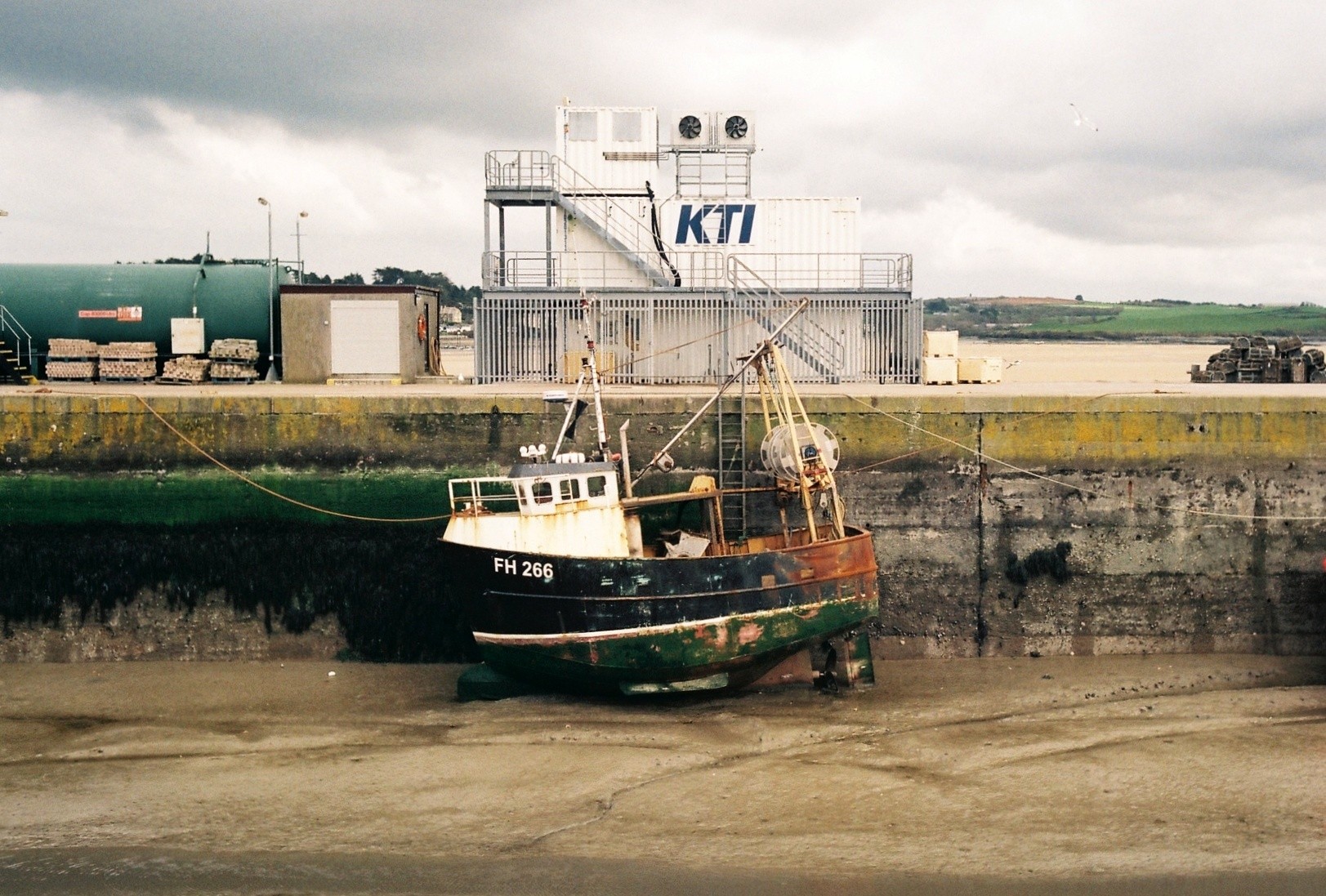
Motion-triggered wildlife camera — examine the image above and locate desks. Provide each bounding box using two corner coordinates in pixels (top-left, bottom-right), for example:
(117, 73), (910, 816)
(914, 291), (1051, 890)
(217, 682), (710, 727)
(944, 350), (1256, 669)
(563, 352), (614, 384)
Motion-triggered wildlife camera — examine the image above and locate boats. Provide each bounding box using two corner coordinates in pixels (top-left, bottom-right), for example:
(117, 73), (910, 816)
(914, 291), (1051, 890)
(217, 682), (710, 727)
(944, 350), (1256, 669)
(432, 283), (881, 704)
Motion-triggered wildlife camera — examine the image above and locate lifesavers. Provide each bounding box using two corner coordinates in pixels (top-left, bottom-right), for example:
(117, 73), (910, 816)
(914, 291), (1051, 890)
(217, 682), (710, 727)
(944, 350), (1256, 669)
(417, 313), (426, 342)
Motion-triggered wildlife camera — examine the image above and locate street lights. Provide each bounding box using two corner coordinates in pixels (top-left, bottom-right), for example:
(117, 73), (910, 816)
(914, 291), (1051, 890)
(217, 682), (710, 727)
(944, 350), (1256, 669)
(296, 211), (308, 286)
(257, 195), (281, 383)
